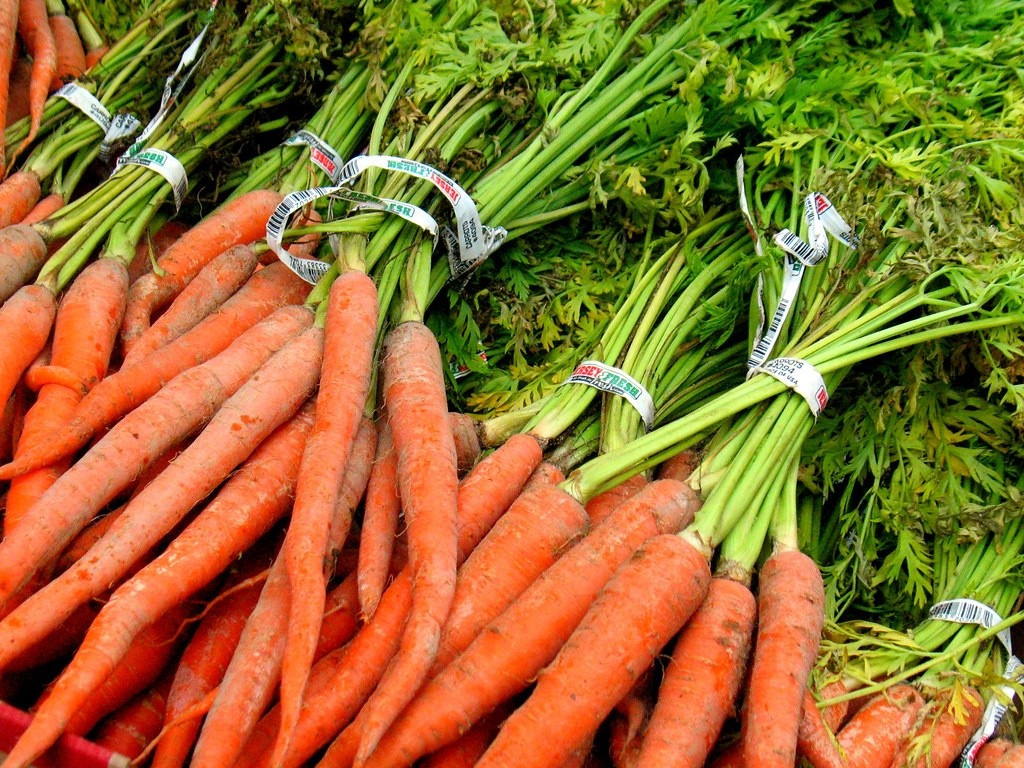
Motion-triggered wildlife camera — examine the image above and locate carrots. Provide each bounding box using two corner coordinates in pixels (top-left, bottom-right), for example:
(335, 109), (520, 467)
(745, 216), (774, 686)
(0, 0), (1024, 768)
